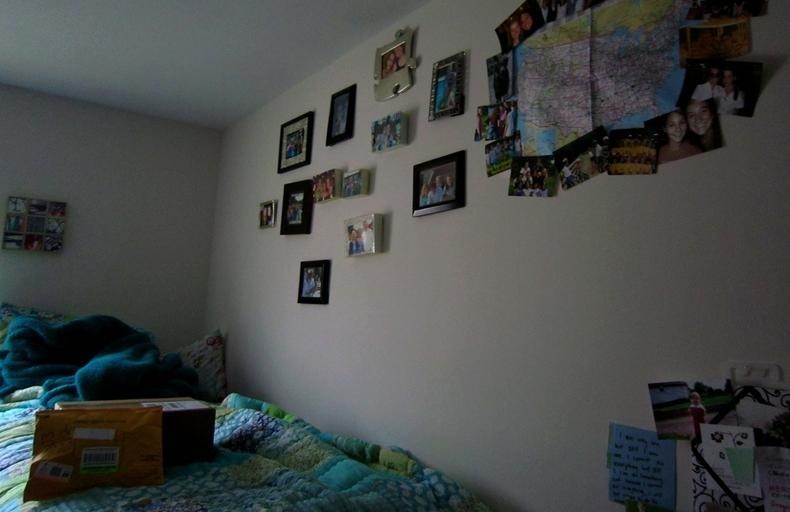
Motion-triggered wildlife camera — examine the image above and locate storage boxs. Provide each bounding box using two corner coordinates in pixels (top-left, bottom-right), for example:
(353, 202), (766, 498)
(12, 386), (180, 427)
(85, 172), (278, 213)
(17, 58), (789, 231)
(54, 397), (215, 464)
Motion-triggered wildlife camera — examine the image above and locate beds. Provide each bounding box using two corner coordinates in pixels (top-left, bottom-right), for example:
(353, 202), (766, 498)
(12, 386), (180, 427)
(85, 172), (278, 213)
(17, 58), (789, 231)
(0, 303), (514, 510)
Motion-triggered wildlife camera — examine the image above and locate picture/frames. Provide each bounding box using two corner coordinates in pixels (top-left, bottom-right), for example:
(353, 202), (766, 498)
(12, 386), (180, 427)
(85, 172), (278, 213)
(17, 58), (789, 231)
(428, 45), (470, 123)
(372, 27), (417, 102)
(297, 261), (331, 305)
(256, 201), (276, 228)
(324, 84), (358, 145)
(413, 150), (467, 218)
(272, 112), (314, 176)
(281, 179), (315, 236)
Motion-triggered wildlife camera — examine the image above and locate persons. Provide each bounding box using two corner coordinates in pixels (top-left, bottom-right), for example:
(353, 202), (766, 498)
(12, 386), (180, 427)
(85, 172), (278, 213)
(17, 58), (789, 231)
(561, 101), (714, 185)
(287, 205), (302, 221)
(474, 58), (520, 164)
(349, 220), (373, 255)
(382, 46), (405, 77)
(689, 1), (749, 114)
(514, 159), (548, 197)
(303, 269), (321, 298)
(444, 65), (456, 108)
(420, 176), (454, 206)
(508, 1), (580, 51)
(688, 391), (705, 435)
(262, 207), (272, 225)
